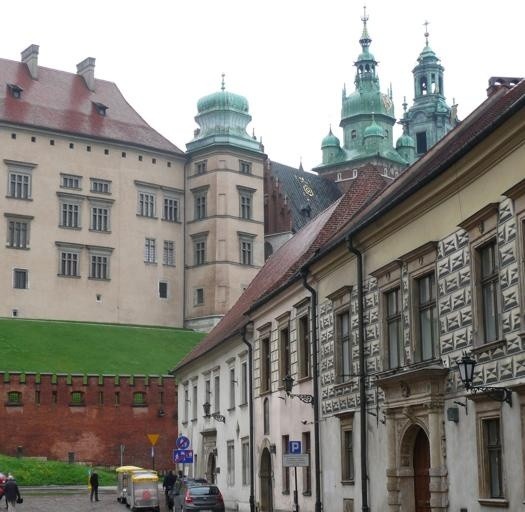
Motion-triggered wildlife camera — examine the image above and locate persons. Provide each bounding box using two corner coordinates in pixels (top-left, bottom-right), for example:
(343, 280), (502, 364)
(0, 472), (20, 512)
(90, 469), (100, 502)
(163, 470), (177, 505)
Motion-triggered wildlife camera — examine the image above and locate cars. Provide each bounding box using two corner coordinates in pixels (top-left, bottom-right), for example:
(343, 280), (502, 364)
(168, 477), (225, 512)
(0, 473), (8, 499)
